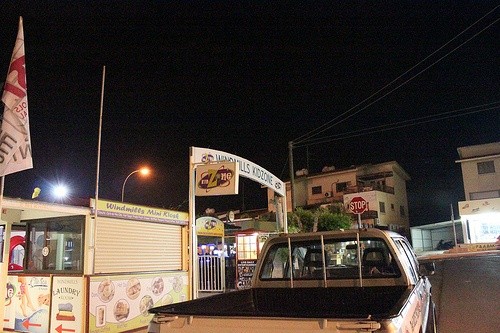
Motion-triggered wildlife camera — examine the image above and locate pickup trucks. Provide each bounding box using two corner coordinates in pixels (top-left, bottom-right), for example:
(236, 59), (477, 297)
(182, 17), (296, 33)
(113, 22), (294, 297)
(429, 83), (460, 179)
(147, 228), (438, 333)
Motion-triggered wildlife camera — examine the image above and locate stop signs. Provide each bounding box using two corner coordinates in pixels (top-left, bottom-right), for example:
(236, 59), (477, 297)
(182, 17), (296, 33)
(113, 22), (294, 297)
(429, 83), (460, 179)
(350, 198), (367, 215)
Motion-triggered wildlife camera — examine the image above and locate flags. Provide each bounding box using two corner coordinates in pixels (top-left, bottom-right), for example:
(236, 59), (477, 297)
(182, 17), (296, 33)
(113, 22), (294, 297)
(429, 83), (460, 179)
(0, 15), (34, 177)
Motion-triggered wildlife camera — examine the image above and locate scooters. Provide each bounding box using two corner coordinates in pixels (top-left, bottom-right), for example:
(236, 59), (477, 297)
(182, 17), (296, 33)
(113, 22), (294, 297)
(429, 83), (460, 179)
(436, 240), (452, 250)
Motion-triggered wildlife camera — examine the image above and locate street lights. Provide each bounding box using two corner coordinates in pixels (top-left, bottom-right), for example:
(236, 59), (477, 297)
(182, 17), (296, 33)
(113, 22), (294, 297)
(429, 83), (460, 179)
(121, 167), (149, 202)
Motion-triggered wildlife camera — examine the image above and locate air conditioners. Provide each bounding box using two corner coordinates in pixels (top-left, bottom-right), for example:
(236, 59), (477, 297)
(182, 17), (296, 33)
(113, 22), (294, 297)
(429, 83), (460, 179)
(388, 223), (400, 232)
(324, 190), (333, 197)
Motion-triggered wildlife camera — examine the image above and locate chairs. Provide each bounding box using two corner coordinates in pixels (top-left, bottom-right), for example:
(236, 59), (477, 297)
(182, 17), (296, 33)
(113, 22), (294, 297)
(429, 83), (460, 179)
(302, 248), (329, 279)
(360, 247), (391, 278)
